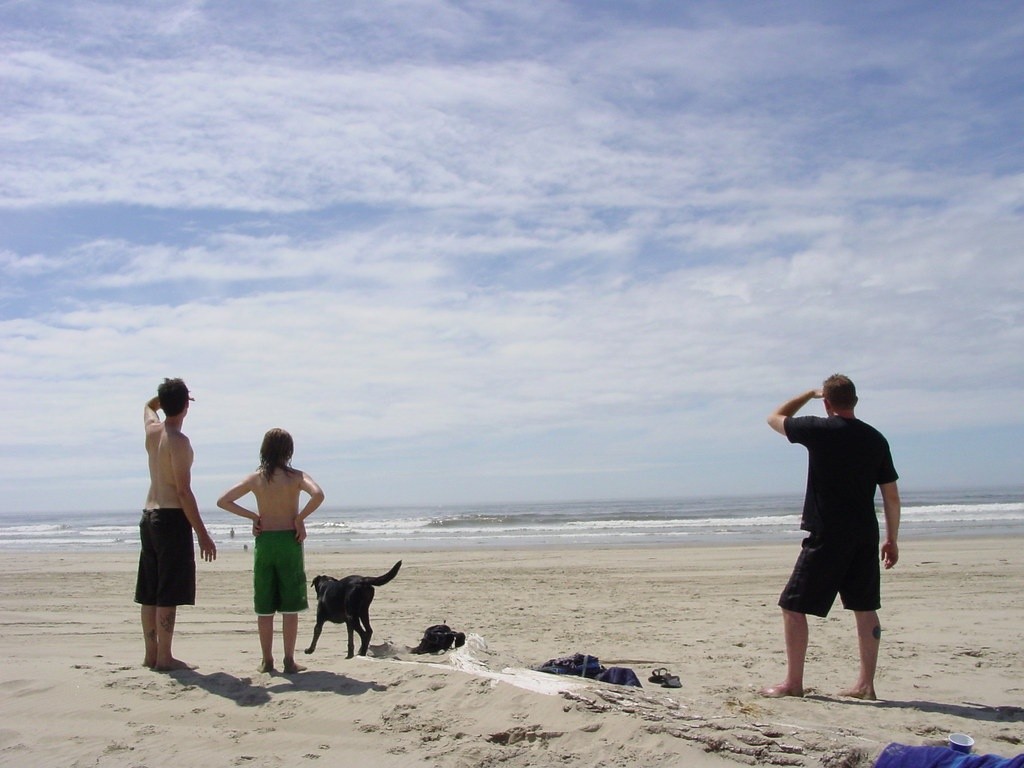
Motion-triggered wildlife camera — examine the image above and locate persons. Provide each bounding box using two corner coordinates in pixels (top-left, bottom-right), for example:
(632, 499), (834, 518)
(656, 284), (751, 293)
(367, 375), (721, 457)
(230, 527), (234, 537)
(134, 377), (216, 670)
(759, 373), (900, 703)
(217, 428), (325, 675)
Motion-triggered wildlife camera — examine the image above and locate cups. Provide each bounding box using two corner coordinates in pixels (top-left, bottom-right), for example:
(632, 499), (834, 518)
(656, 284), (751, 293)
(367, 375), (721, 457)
(948, 733), (975, 755)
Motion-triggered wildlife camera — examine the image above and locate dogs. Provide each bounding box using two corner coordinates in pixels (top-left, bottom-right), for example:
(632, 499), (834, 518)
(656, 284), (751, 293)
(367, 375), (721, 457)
(304, 558), (402, 660)
